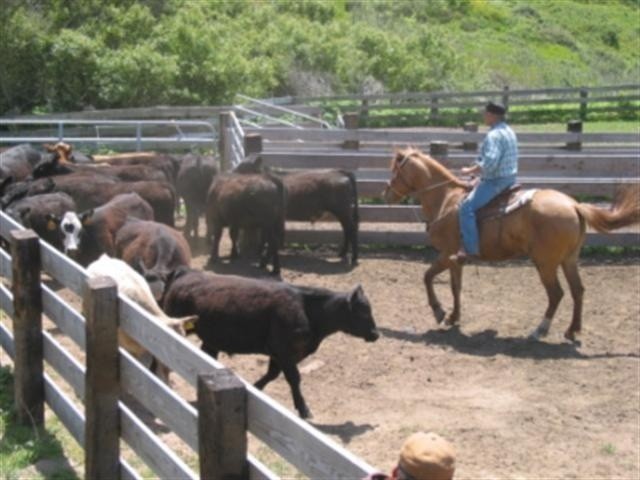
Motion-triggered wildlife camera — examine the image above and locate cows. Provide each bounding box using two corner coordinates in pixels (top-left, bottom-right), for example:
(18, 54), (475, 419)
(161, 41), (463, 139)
(82, 252), (199, 386)
(150, 262), (379, 421)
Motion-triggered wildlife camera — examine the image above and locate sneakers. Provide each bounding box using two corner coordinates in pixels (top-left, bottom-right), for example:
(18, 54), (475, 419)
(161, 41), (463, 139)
(448, 250), (483, 266)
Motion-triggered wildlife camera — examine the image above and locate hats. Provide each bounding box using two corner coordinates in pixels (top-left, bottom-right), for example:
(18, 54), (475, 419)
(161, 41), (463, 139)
(476, 98), (511, 121)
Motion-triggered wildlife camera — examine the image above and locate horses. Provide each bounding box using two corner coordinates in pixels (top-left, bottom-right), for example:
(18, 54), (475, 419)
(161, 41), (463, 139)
(204, 166), (280, 277)
(3, 143), (216, 300)
(262, 168), (359, 269)
(382, 142), (639, 344)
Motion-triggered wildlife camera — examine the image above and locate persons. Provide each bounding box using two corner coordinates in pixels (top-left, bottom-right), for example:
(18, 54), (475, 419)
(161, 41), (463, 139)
(447, 100), (521, 262)
